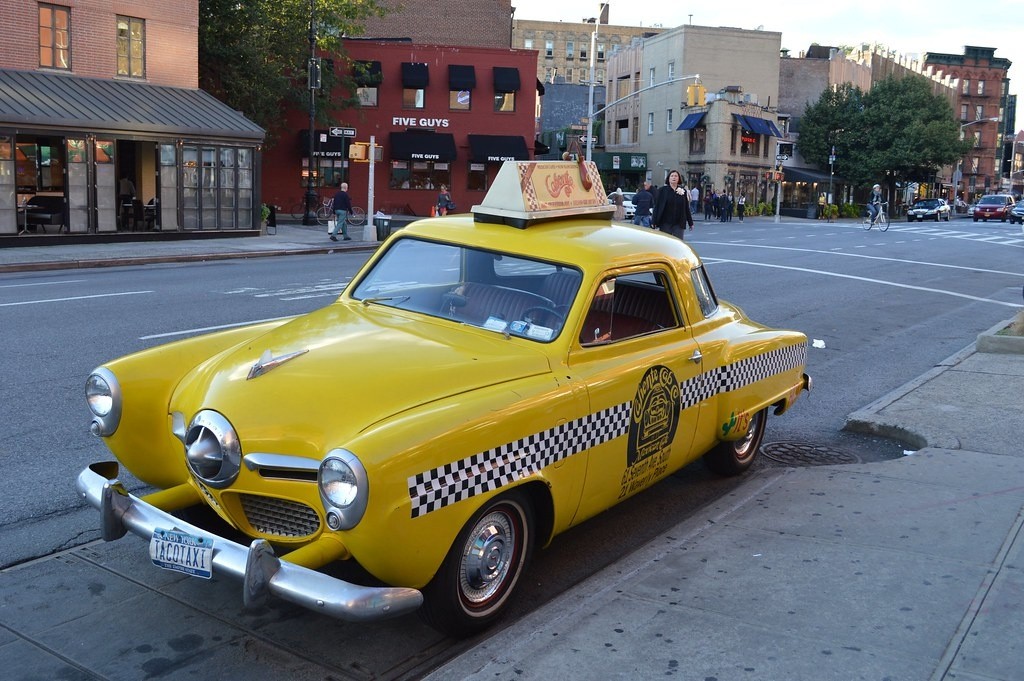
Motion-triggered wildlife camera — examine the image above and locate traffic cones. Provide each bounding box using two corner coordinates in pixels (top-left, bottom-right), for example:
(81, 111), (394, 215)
(430, 206), (435, 217)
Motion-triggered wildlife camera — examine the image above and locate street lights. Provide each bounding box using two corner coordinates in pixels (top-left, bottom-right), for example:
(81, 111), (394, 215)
(950, 117), (998, 215)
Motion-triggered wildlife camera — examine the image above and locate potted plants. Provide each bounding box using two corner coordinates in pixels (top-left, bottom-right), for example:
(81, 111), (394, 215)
(259, 206), (271, 235)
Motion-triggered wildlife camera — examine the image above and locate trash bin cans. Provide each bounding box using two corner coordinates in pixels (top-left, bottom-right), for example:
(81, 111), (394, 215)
(374, 216), (392, 240)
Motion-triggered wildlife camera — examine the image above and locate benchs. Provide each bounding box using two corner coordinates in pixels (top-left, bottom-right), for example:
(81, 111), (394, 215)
(541, 269), (674, 329)
(18, 196), (66, 234)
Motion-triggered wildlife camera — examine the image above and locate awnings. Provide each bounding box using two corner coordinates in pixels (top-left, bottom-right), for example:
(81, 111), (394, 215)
(537, 78), (545, 96)
(390, 132), (457, 161)
(352, 60), (382, 84)
(493, 67), (520, 91)
(449, 64), (476, 88)
(402, 62), (429, 87)
(468, 135), (530, 162)
(676, 112), (705, 131)
(735, 115), (782, 138)
(300, 129), (351, 158)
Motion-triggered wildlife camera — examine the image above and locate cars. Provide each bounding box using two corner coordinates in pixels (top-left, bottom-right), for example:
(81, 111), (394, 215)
(1010, 200), (1024, 224)
(950, 201), (969, 213)
(74, 158), (813, 641)
(967, 205), (975, 217)
(907, 197), (950, 222)
(607, 190), (652, 219)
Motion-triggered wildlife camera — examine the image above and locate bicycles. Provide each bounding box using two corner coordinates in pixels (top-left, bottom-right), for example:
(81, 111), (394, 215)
(861, 202), (890, 231)
(291, 194), (366, 226)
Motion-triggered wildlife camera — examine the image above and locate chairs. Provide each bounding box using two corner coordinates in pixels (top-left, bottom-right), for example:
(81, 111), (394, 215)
(443, 281), (555, 326)
(550, 305), (657, 344)
(117, 199), (156, 233)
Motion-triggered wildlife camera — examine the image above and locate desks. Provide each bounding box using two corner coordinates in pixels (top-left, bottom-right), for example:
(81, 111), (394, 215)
(122, 204), (156, 232)
(18, 204), (37, 235)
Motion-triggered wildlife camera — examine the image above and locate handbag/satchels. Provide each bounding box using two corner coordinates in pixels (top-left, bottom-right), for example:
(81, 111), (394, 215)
(327, 216), (343, 234)
(436, 210), (439, 217)
(447, 202), (456, 210)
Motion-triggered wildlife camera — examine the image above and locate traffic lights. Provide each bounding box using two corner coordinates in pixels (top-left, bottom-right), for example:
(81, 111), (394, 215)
(697, 86), (707, 106)
(765, 171), (774, 180)
(775, 172), (784, 182)
(368, 146), (383, 162)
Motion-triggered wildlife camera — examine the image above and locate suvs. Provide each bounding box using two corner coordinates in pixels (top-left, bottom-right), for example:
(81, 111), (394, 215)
(973, 194), (1016, 223)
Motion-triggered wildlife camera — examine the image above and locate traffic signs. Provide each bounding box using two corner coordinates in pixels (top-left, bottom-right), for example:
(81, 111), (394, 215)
(329, 127), (356, 138)
(776, 154), (789, 160)
(579, 136), (598, 144)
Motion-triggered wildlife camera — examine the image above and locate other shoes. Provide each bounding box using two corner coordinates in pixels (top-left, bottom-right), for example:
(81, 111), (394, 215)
(344, 237), (351, 240)
(871, 222), (874, 226)
(330, 236), (338, 241)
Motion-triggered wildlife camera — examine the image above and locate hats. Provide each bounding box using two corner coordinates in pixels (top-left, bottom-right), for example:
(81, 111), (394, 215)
(873, 184), (879, 189)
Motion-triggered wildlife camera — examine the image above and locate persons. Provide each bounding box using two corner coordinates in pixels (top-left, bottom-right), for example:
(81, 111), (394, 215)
(634, 181), (658, 227)
(651, 170), (694, 240)
(818, 192), (827, 220)
(401, 178), (409, 188)
(687, 184), (699, 214)
(703, 188), (735, 222)
(330, 183), (353, 241)
(436, 184), (451, 215)
(612, 188), (624, 221)
(119, 170), (135, 214)
(424, 179), (434, 189)
(737, 193), (746, 223)
(866, 184), (885, 224)
